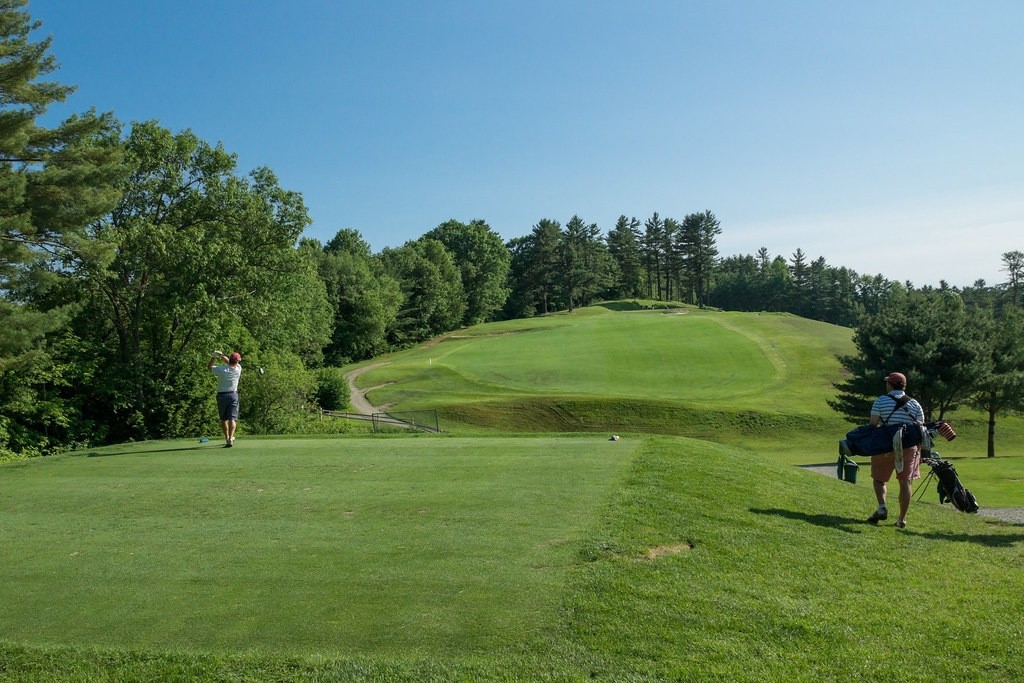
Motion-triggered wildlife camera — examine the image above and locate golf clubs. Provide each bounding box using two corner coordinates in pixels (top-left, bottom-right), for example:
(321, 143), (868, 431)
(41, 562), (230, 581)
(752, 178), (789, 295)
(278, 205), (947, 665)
(911, 450), (942, 502)
(219, 354), (264, 374)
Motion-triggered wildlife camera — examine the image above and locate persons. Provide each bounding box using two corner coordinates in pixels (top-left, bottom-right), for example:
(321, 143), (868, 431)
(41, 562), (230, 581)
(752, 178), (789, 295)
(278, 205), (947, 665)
(208, 351), (241, 447)
(866, 373), (924, 528)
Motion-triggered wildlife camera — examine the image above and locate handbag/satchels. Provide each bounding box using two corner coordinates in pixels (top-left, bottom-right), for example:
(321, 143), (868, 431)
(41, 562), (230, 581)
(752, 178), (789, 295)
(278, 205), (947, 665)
(846, 424), (922, 456)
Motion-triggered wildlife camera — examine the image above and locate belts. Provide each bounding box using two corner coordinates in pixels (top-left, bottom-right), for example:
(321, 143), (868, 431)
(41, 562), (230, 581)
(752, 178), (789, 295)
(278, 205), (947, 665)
(218, 391), (237, 394)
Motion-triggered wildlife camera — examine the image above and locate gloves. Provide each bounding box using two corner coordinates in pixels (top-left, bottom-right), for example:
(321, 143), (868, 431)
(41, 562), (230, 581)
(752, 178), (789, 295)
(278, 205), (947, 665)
(214, 351), (222, 358)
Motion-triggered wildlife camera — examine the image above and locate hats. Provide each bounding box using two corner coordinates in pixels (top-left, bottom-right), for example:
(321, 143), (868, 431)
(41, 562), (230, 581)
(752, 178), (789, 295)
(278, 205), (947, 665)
(230, 353), (241, 360)
(885, 373), (906, 390)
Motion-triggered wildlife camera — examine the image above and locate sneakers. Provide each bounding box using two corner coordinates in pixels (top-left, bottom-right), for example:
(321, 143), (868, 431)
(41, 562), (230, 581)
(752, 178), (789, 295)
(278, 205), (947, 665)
(895, 520), (905, 527)
(226, 437), (235, 446)
(869, 507), (887, 524)
